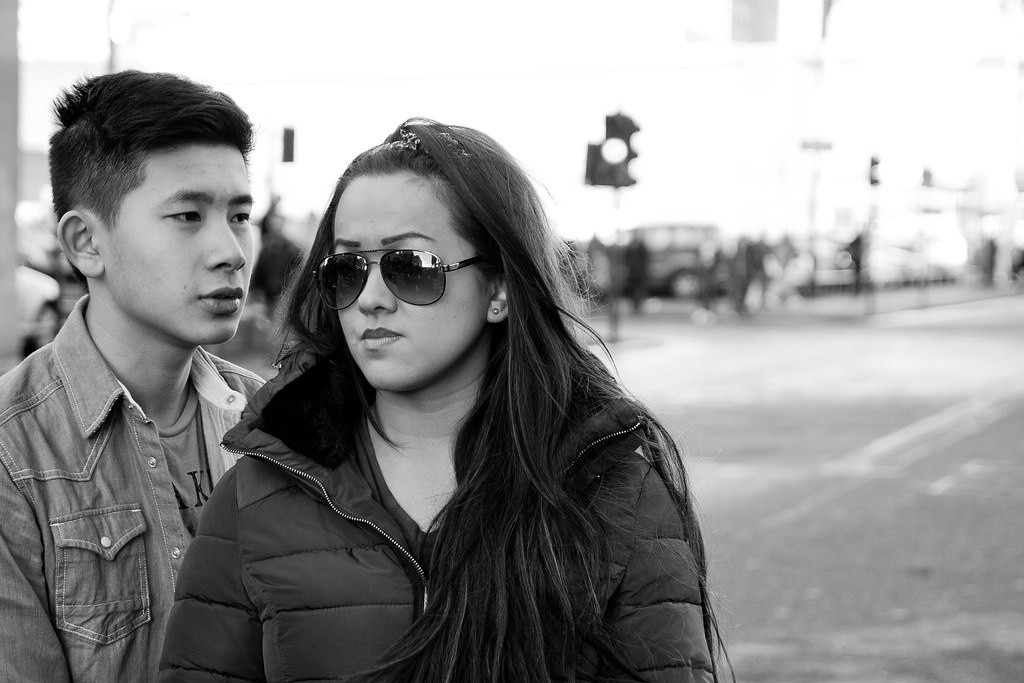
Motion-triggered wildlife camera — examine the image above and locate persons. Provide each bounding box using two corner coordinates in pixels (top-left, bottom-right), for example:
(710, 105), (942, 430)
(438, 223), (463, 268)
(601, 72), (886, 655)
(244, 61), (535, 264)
(624, 226), (652, 317)
(845, 231), (870, 299)
(979, 241), (998, 283)
(0, 68), (265, 683)
(159, 113), (743, 683)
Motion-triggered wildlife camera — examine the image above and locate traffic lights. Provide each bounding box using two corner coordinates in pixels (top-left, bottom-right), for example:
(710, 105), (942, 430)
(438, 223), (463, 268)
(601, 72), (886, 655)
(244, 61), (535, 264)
(584, 143), (604, 185)
(604, 115), (640, 189)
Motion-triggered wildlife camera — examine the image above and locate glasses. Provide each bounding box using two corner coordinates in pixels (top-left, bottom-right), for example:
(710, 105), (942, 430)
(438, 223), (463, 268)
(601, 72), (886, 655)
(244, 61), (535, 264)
(313, 242), (486, 311)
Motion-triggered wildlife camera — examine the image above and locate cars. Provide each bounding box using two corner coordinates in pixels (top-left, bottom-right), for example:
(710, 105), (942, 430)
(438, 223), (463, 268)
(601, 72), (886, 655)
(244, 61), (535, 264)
(559, 201), (978, 313)
